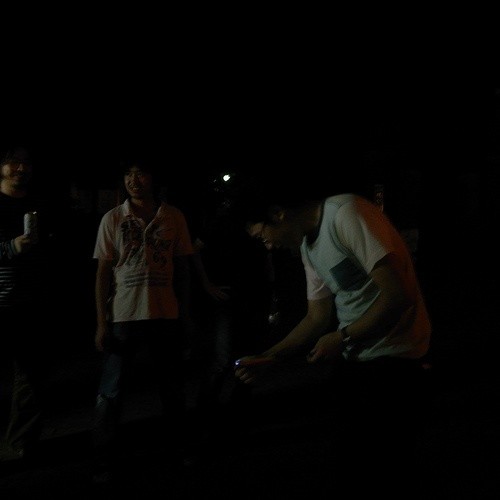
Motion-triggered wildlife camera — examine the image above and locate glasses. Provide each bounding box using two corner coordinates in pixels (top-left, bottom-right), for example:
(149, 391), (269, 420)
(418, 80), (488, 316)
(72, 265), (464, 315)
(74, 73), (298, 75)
(259, 222), (271, 244)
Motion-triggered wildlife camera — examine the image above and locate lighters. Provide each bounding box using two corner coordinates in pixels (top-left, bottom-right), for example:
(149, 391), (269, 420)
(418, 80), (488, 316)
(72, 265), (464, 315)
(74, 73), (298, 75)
(233, 358), (274, 373)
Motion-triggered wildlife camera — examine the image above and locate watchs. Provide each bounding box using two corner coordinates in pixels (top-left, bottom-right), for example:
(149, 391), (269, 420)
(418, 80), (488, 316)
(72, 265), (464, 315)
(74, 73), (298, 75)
(341, 326), (355, 346)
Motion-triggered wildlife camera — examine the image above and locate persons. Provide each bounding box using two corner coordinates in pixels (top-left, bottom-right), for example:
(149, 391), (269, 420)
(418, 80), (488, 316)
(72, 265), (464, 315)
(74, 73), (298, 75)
(88, 155), (231, 485)
(233, 193), (433, 500)
(1, 137), (62, 460)
